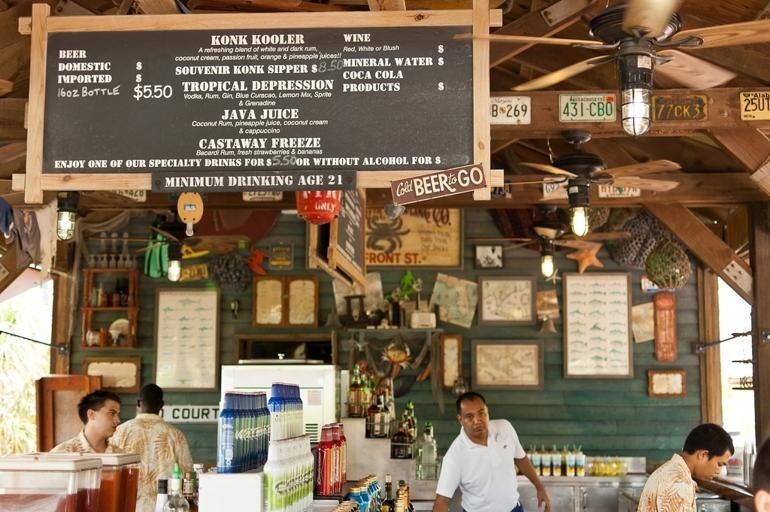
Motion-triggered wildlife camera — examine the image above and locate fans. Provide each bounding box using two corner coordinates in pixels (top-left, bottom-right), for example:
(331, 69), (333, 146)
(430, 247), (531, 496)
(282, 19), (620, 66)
(452, 3), (770, 94)
(465, 206), (633, 251)
(503, 129), (683, 192)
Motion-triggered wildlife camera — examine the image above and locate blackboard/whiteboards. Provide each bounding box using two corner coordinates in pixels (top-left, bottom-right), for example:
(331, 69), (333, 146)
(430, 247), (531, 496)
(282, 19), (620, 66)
(42, 26), (475, 174)
(317, 190), (365, 285)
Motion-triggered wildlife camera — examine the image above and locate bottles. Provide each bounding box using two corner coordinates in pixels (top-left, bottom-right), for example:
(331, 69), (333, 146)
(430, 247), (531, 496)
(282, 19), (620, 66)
(271, 383), (304, 438)
(112, 278), (121, 306)
(349, 474), (381, 512)
(156, 479), (168, 512)
(339, 423), (346, 482)
(368, 392), (380, 436)
(379, 391), (390, 437)
(394, 480), (414, 512)
(318, 426), (333, 496)
(97, 280), (103, 305)
(263, 435), (314, 512)
(173, 463), (181, 489)
(423, 429), (437, 478)
(220, 392), (269, 474)
(392, 423), (409, 458)
(332, 423), (341, 493)
(381, 475), (395, 512)
(183, 472), (194, 504)
(349, 363), (374, 417)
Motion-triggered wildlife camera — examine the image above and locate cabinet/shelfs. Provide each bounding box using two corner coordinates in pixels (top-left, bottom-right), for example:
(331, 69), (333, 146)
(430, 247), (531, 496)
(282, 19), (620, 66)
(79, 268), (139, 351)
(517, 482), (646, 512)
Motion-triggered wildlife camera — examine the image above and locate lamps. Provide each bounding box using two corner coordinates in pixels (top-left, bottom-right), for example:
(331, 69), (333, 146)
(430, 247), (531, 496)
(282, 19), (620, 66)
(56, 191), (79, 241)
(617, 54), (653, 137)
(567, 186), (590, 236)
(166, 243), (182, 282)
(540, 243), (555, 277)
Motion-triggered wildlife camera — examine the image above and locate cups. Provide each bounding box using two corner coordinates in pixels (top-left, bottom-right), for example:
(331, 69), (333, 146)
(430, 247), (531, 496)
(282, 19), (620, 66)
(526, 452), (585, 477)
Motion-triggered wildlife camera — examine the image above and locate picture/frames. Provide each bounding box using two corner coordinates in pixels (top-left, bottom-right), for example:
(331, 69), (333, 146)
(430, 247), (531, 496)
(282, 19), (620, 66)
(441, 334), (463, 390)
(268, 242), (295, 272)
(152, 287), (222, 394)
(478, 275), (537, 327)
(562, 272), (635, 381)
(365, 189), (466, 272)
(647, 369), (687, 398)
(285, 275), (319, 328)
(80, 356), (142, 393)
(470, 339), (545, 392)
(251, 275), (285, 329)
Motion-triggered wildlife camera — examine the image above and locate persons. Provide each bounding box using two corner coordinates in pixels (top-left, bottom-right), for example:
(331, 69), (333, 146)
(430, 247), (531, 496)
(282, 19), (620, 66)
(47, 388), (128, 454)
(752, 435), (770, 512)
(431, 391), (552, 512)
(633, 422), (736, 511)
(106, 382), (194, 512)
